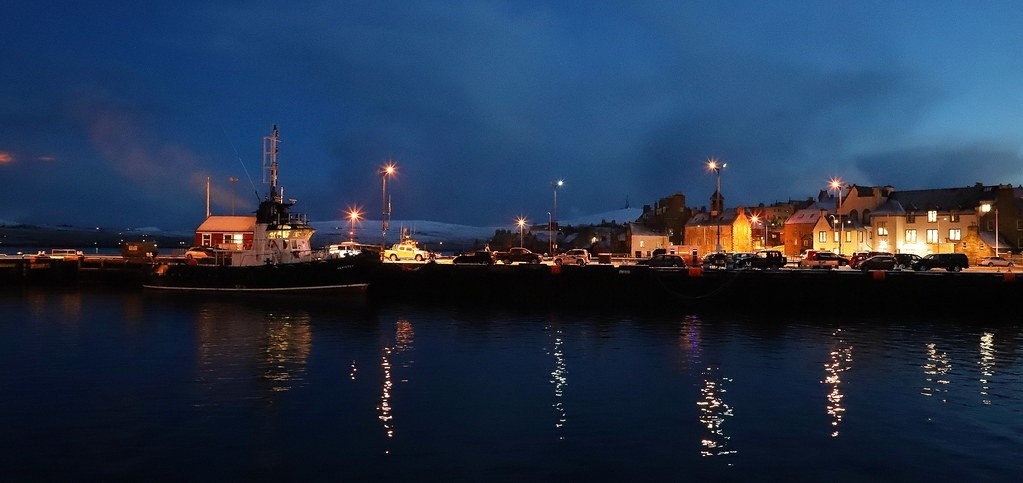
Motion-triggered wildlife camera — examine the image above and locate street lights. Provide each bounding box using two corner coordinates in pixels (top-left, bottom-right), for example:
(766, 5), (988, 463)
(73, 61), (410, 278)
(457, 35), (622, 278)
(981, 203), (999, 257)
(382, 166), (396, 249)
(708, 161), (720, 254)
(517, 218), (525, 249)
(554, 180), (563, 255)
(828, 178), (841, 254)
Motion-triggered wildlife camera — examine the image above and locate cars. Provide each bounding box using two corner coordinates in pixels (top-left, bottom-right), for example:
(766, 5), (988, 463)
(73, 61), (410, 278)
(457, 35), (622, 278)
(702, 250), (788, 271)
(185, 245), (210, 259)
(977, 256), (1015, 267)
(798, 250), (969, 275)
(648, 254), (686, 268)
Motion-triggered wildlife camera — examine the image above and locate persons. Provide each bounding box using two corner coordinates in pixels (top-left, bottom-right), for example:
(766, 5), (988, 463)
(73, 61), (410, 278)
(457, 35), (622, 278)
(483, 243), (491, 252)
(186, 258), (199, 267)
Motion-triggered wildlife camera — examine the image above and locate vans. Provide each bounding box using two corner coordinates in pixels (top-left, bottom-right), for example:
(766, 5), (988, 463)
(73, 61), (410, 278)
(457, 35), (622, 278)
(329, 244), (362, 260)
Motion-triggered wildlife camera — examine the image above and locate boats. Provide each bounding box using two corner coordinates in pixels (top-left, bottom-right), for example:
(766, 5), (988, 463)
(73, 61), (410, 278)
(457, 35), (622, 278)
(139, 123), (370, 303)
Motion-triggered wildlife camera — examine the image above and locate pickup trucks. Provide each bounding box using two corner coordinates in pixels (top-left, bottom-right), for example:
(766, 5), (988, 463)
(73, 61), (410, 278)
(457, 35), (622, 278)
(495, 247), (544, 266)
(383, 244), (429, 262)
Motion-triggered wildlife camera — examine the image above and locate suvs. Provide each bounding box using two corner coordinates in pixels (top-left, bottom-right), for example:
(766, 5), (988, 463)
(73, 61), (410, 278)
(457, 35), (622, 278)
(554, 249), (592, 266)
